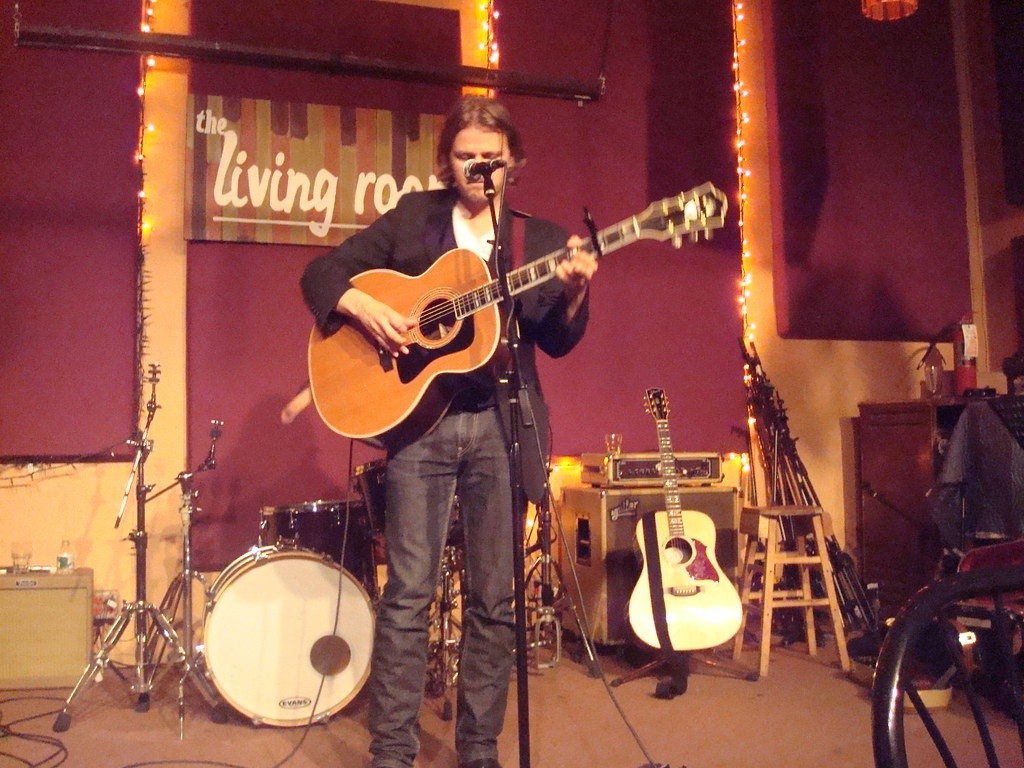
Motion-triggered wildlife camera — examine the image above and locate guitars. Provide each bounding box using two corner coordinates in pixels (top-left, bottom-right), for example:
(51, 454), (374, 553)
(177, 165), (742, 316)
(623, 385), (744, 658)
(306, 179), (729, 452)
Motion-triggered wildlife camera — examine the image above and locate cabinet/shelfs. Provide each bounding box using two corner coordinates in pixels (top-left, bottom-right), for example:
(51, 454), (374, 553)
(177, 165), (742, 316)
(858, 404), (963, 636)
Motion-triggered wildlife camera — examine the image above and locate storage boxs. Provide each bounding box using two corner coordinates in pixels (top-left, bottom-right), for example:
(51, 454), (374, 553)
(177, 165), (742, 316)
(561, 485), (736, 661)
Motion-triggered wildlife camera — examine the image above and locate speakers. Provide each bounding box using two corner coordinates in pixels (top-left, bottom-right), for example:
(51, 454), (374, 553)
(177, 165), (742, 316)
(0, 567), (94, 700)
(558, 484), (740, 648)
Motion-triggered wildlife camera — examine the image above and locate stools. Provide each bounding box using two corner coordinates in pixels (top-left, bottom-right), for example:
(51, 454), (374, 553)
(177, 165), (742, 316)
(733, 504), (851, 675)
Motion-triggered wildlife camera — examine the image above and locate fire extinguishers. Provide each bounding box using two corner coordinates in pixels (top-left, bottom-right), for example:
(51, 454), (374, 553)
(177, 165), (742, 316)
(916, 310), (978, 399)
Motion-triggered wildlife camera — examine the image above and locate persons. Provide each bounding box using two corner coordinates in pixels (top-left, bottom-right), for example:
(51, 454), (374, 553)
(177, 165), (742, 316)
(299, 94), (598, 768)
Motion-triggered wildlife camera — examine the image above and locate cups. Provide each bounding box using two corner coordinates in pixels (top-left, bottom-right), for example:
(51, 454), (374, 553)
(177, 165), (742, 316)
(604, 433), (622, 456)
(924, 365), (943, 406)
(11, 551), (30, 575)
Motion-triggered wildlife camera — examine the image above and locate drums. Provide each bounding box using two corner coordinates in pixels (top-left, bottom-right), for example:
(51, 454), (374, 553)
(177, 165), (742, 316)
(202, 543), (376, 728)
(352, 456), (463, 565)
(257, 497), (381, 613)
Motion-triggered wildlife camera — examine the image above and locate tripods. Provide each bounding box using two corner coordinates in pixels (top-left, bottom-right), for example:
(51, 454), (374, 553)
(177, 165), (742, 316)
(52, 363), (228, 733)
(737, 336), (886, 654)
(527, 487), (603, 679)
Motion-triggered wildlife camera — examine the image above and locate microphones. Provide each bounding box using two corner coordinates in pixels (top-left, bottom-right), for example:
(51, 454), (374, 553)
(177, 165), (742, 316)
(462, 158), (507, 182)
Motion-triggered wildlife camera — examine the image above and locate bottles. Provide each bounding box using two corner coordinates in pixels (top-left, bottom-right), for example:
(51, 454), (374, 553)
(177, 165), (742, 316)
(55, 539), (75, 576)
